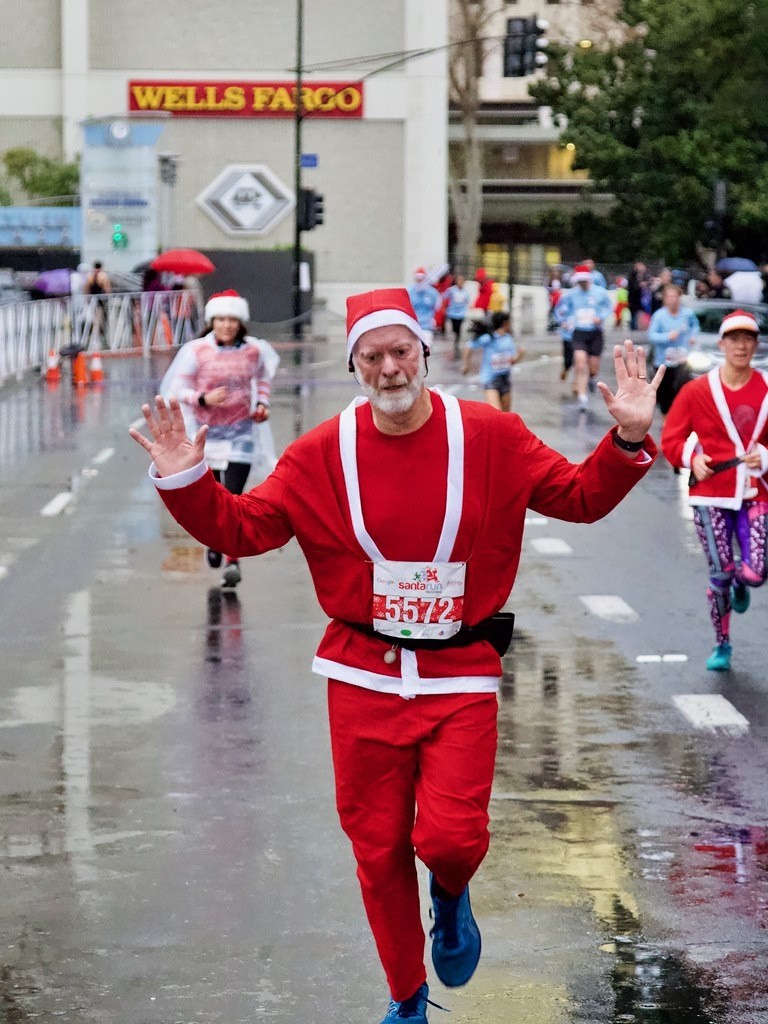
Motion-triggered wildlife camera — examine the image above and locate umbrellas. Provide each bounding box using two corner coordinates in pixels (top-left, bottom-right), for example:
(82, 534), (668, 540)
(148, 248), (216, 274)
(713, 257), (760, 271)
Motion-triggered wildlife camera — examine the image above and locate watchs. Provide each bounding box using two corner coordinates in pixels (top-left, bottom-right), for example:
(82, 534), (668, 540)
(198, 393), (205, 407)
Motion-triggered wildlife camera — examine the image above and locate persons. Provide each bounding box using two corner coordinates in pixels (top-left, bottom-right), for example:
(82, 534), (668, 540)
(460, 311), (526, 411)
(129, 286), (666, 1024)
(661, 309), (768, 670)
(157, 288), (281, 588)
(407, 257), (768, 411)
(83, 261), (111, 336)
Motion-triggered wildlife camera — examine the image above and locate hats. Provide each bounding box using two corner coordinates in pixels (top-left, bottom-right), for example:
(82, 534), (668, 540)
(345, 288), (431, 364)
(475, 268), (487, 282)
(616, 277), (625, 286)
(719, 310), (759, 340)
(571, 266), (591, 284)
(203, 288), (249, 329)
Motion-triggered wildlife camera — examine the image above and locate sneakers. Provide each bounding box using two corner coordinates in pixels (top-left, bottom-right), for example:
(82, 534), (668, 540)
(381, 982), (451, 1024)
(730, 555), (751, 614)
(703, 643), (731, 671)
(425, 872), (481, 989)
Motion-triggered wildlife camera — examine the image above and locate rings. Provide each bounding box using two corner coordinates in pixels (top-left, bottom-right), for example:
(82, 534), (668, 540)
(638, 376), (646, 379)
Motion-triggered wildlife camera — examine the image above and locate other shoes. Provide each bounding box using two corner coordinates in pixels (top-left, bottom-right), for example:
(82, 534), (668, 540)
(578, 399), (587, 410)
(589, 378), (598, 392)
(206, 546), (222, 570)
(222, 562), (241, 587)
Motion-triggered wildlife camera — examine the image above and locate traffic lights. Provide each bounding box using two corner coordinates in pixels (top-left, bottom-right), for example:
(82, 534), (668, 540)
(299, 189), (324, 230)
(523, 14), (549, 69)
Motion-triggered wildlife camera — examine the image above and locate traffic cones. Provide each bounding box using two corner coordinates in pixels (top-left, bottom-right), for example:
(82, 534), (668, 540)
(46, 348), (60, 380)
(90, 352), (104, 381)
(73, 353), (89, 383)
(153, 313), (172, 344)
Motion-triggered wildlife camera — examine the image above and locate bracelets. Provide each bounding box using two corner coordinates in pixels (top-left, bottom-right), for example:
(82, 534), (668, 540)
(613, 433), (644, 452)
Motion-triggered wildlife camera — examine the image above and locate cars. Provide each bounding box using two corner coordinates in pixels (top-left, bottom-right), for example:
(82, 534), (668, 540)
(645, 299), (768, 383)
